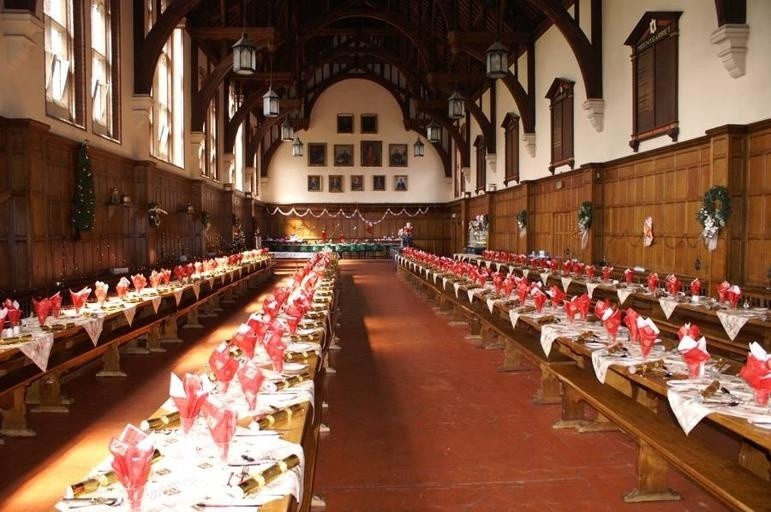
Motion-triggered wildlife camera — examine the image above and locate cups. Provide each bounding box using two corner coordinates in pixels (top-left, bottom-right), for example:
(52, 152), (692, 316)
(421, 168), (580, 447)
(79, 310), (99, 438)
(0, 298), (86, 338)
(180, 314), (290, 458)
(120, 476), (148, 511)
(512, 259), (769, 407)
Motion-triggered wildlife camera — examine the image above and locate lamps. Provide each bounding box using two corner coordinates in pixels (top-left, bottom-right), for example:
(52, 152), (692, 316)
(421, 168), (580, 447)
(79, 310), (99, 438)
(233, 2), (305, 158)
(414, 0), (511, 157)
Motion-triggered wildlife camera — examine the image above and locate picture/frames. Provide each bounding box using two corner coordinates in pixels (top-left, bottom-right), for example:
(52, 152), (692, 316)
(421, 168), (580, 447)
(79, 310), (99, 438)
(306, 113), (409, 191)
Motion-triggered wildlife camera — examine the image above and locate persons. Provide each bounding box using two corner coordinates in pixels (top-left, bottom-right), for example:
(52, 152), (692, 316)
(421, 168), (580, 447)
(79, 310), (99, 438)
(307, 140), (408, 192)
(404, 220), (416, 247)
